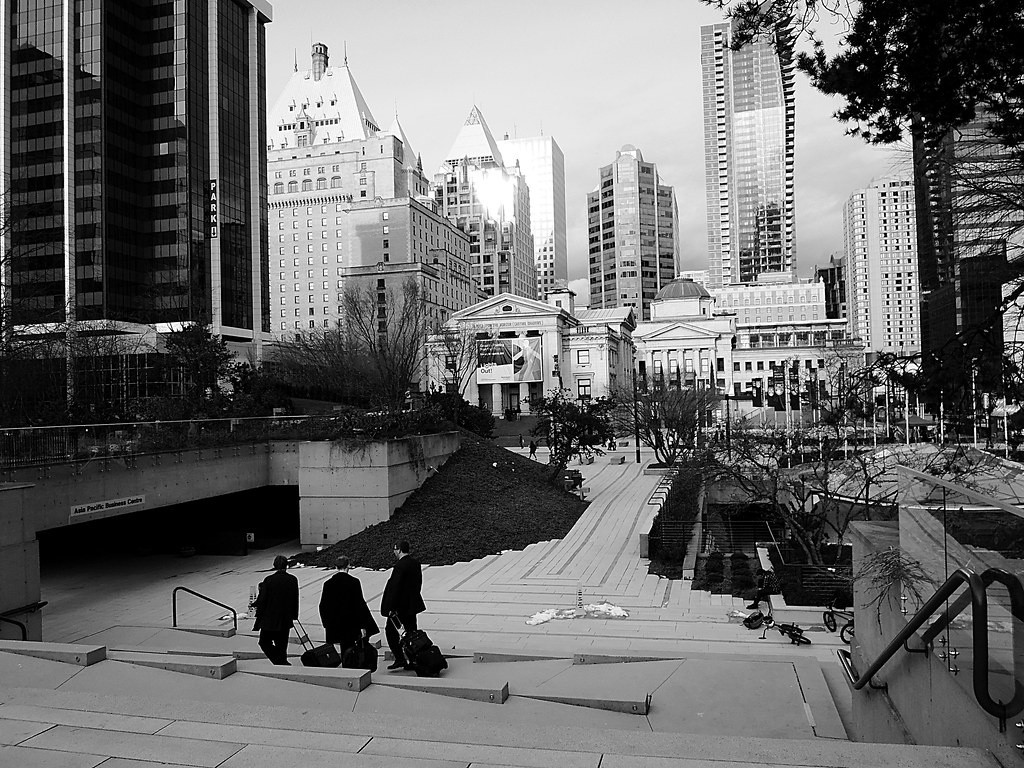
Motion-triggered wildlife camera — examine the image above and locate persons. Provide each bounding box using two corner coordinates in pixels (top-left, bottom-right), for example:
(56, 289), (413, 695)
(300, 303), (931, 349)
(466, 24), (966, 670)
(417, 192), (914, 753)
(746, 592), (759, 609)
(756, 590), (770, 618)
(250, 554), (300, 665)
(516, 404), (521, 421)
(512, 408), (516, 416)
(380, 539), (428, 671)
(518, 433), (525, 449)
(505, 406), (510, 419)
(766, 595), (772, 617)
(712, 430), (718, 447)
(529, 441), (538, 461)
(770, 432), (776, 450)
(318, 556), (379, 668)
(720, 429), (725, 446)
(779, 432), (786, 452)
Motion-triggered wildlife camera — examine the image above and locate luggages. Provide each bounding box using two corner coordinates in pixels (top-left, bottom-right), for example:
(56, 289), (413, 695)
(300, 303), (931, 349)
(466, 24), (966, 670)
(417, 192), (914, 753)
(390, 615), (449, 676)
(344, 637), (378, 673)
(292, 618), (341, 667)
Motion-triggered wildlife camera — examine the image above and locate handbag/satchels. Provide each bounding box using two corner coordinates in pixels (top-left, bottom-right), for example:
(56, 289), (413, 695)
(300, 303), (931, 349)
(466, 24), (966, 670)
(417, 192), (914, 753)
(398, 629), (433, 655)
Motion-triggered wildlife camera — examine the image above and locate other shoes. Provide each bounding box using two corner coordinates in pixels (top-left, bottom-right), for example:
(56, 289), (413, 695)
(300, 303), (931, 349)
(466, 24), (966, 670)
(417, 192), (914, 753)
(387, 658), (417, 671)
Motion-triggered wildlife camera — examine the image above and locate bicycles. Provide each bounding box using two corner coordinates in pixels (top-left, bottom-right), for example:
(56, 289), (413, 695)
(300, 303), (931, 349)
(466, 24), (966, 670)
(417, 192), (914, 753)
(840, 611), (855, 644)
(822, 597), (855, 632)
(759, 604), (812, 646)
